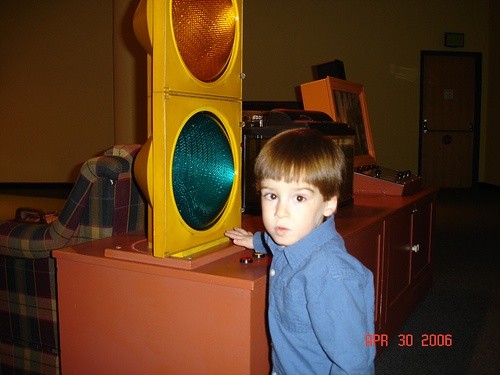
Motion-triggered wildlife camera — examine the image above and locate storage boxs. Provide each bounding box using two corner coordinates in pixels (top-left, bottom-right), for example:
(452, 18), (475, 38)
(242, 121), (358, 218)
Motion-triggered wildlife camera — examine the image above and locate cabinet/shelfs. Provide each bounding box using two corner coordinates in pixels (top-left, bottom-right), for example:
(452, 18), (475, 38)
(52, 175), (442, 375)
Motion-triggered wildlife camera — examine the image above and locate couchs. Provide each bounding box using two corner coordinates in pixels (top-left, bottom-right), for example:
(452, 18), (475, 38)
(1, 143), (143, 374)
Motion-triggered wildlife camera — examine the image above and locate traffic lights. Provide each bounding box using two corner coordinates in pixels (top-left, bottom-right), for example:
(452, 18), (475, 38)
(133, 0), (246, 259)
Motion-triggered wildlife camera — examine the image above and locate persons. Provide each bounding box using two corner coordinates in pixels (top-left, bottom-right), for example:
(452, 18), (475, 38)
(224, 129), (378, 375)
